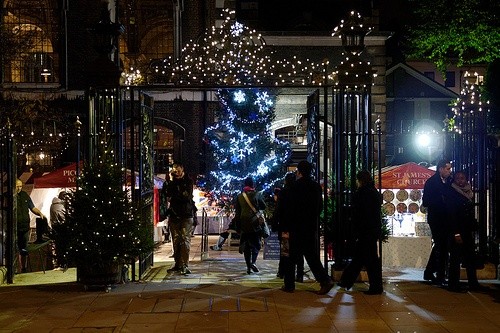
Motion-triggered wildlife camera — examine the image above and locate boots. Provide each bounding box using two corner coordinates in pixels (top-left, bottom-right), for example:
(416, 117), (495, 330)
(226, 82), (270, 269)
(21, 255), (29, 272)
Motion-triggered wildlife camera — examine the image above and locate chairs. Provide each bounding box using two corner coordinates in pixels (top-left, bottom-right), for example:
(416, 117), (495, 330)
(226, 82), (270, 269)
(227, 229), (241, 252)
(21, 218), (52, 274)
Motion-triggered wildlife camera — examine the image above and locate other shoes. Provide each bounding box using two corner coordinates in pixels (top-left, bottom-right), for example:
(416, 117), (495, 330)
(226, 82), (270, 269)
(364, 287), (383, 294)
(167, 266), (182, 273)
(424, 271), (437, 280)
(247, 269), (251, 274)
(435, 276), (448, 284)
(336, 282), (353, 290)
(317, 281), (334, 294)
(447, 286), (466, 293)
(468, 285), (490, 292)
(295, 277), (303, 282)
(251, 263), (259, 272)
(283, 288), (293, 292)
(181, 268), (186, 274)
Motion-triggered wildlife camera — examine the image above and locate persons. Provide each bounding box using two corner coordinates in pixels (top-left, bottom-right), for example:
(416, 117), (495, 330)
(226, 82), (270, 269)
(16, 179), (46, 273)
(162, 161), (240, 275)
(272, 160), (492, 295)
(50, 191), (78, 270)
(235, 179), (267, 273)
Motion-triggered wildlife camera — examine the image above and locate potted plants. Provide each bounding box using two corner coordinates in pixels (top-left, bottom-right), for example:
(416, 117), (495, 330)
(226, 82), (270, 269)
(47, 147), (158, 291)
(319, 146), (392, 283)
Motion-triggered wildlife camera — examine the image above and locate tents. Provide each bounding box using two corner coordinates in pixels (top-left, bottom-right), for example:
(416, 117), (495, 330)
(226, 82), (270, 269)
(34, 160), (165, 189)
(371, 161), (436, 189)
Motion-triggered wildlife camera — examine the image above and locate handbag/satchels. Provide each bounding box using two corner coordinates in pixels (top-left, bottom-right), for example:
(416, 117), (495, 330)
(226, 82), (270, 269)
(258, 214), (270, 239)
